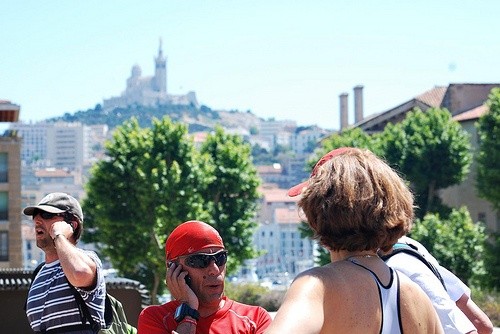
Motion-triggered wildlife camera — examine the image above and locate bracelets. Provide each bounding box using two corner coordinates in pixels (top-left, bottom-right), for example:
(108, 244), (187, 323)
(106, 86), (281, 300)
(178, 317), (198, 325)
(51, 234), (69, 248)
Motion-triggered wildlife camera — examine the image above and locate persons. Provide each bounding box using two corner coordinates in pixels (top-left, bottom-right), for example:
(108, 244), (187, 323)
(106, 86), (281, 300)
(135, 220), (272, 334)
(23, 191), (106, 334)
(257, 145), (495, 334)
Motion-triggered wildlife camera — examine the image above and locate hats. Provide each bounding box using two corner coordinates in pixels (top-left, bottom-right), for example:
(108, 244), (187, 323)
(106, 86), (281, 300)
(165, 220), (225, 261)
(288, 147), (371, 197)
(24, 192), (83, 224)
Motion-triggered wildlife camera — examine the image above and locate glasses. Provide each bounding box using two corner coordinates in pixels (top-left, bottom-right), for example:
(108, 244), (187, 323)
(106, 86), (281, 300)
(33, 210), (72, 221)
(170, 250), (227, 268)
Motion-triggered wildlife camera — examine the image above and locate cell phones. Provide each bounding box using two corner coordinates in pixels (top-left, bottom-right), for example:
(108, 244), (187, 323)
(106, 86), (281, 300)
(168, 261), (191, 286)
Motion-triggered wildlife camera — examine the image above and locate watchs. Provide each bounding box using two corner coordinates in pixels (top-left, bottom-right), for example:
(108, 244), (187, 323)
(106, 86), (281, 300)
(173, 303), (202, 323)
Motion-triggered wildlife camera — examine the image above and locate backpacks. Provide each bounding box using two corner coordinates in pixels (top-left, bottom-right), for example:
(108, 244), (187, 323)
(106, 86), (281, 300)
(23, 262), (138, 334)
